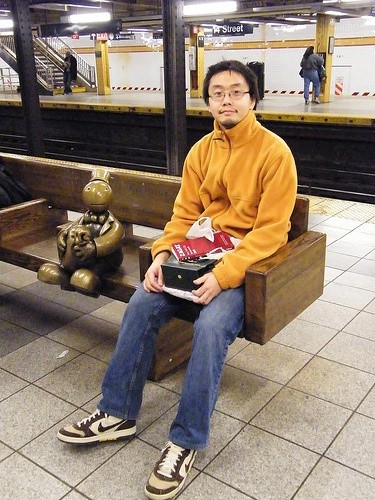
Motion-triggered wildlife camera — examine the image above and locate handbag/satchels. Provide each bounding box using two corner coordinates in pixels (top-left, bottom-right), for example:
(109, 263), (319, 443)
(317, 65), (327, 82)
(162, 217), (243, 300)
(299, 68), (304, 78)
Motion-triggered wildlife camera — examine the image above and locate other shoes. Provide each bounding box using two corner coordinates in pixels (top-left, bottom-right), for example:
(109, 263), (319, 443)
(305, 99), (310, 105)
(314, 96), (320, 104)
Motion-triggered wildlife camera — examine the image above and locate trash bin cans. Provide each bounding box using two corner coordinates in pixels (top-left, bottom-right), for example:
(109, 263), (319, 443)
(246, 61), (264, 99)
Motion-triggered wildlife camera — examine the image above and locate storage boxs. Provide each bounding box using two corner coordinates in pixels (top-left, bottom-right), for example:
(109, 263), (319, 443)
(161, 256), (218, 291)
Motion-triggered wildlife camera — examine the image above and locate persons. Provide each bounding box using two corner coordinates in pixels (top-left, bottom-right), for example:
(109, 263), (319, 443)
(37, 168), (126, 298)
(299, 46), (324, 105)
(57, 60), (298, 500)
(63, 51), (77, 95)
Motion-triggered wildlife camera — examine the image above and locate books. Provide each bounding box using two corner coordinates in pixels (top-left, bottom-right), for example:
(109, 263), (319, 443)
(171, 230), (234, 263)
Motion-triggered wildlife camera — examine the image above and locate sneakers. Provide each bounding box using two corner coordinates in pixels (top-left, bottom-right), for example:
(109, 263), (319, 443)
(144, 441), (197, 500)
(56, 408), (136, 445)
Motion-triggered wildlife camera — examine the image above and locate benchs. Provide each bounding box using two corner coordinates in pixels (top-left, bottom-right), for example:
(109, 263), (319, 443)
(0, 154), (327, 380)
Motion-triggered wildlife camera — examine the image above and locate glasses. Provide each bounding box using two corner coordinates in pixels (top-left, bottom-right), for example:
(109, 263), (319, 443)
(209, 89), (250, 101)
